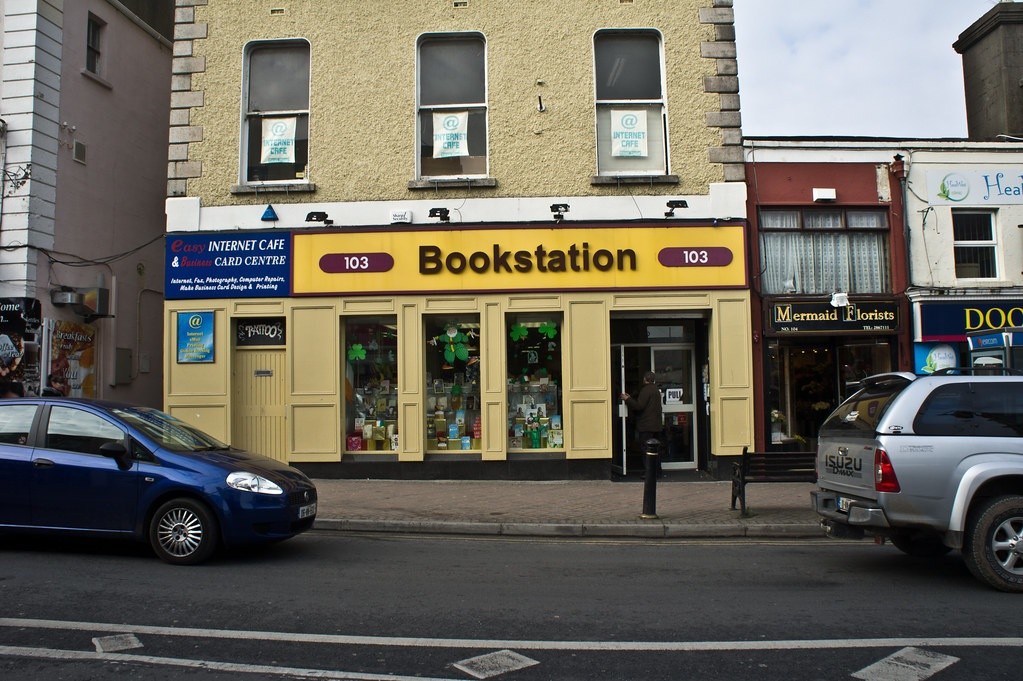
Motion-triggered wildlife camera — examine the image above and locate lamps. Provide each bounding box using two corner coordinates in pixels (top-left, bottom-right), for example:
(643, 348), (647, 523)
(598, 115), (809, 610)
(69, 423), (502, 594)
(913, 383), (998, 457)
(665, 200), (688, 219)
(830, 292), (851, 307)
(305, 212), (333, 226)
(550, 204), (570, 223)
(428, 208), (450, 223)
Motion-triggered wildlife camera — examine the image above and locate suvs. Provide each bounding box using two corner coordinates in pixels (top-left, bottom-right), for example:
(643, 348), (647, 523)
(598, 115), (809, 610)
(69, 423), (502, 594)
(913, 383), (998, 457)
(814, 368), (1023, 591)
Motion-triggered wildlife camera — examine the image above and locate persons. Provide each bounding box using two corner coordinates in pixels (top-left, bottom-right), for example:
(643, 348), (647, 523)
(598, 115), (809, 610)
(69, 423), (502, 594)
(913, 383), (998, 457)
(1, 374), (66, 400)
(620, 372), (664, 480)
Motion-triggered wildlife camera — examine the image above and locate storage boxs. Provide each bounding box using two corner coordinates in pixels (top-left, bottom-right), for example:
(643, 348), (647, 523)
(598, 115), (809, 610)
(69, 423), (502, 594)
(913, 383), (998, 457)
(347, 418), (563, 449)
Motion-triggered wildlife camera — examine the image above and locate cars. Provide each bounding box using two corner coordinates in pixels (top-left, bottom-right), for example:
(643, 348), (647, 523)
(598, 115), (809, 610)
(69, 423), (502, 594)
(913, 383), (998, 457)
(0, 397), (318, 562)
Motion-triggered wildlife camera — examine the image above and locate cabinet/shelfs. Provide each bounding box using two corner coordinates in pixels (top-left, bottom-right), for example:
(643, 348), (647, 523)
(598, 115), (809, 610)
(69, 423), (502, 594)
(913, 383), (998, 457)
(347, 381), (557, 416)
